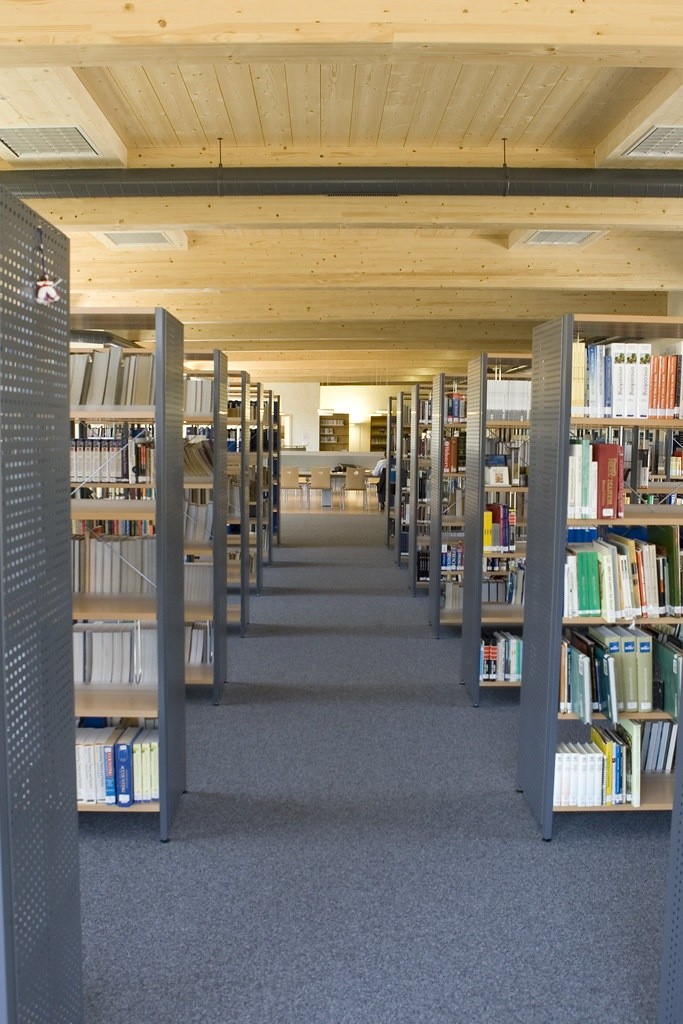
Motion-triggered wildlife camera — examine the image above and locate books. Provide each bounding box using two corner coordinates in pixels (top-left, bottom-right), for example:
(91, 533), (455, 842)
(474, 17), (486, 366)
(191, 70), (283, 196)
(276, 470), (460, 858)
(72, 349), (274, 807)
(389, 344), (683, 808)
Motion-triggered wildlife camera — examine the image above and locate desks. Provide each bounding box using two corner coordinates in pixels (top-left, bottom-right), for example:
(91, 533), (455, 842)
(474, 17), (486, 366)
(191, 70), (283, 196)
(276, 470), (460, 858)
(297, 470), (379, 504)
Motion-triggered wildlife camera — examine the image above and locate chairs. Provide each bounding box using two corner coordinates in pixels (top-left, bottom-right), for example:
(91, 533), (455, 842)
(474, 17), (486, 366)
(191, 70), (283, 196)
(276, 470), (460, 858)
(376, 468), (407, 510)
(280, 464), (382, 512)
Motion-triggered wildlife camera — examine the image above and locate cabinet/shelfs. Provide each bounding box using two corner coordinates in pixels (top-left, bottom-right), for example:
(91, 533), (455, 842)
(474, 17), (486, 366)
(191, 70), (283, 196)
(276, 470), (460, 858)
(370, 314), (681, 844)
(320, 414), (349, 452)
(0, 188), (283, 1024)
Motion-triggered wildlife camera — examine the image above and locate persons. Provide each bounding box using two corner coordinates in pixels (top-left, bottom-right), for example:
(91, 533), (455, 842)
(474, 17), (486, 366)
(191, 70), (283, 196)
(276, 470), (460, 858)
(372, 452), (387, 477)
